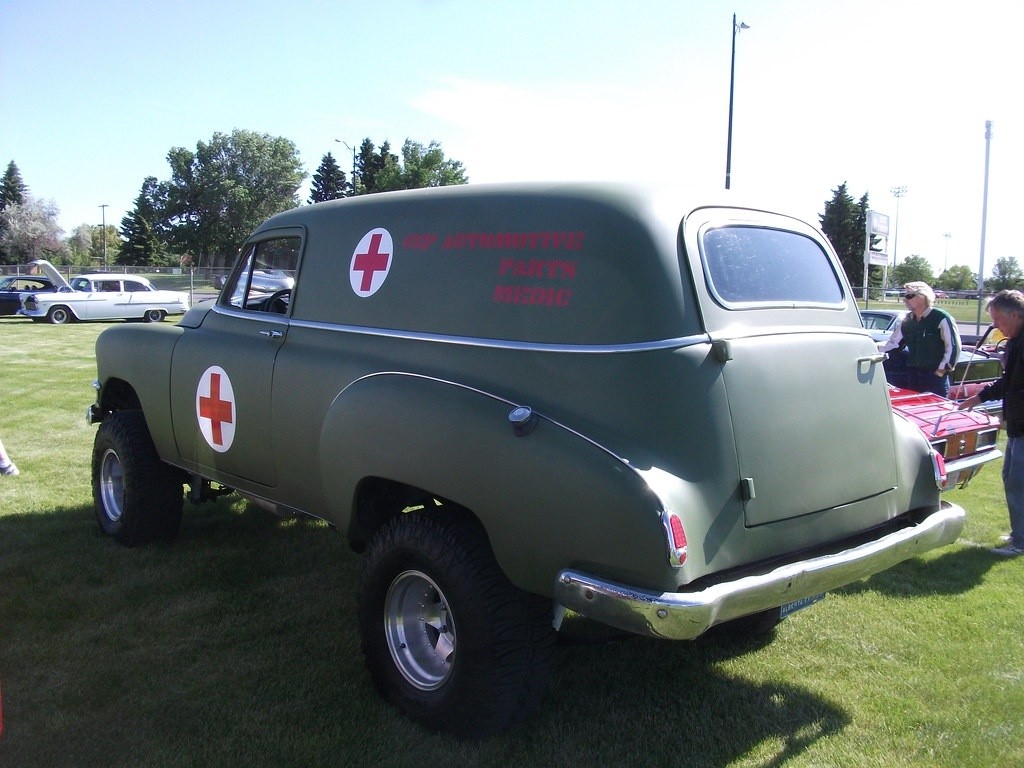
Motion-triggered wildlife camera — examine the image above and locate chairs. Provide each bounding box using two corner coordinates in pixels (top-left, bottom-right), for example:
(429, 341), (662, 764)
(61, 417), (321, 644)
(32, 285), (37, 290)
(25, 285), (30, 290)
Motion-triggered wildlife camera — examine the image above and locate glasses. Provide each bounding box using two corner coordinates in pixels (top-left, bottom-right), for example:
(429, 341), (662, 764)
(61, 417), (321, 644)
(904, 293), (916, 300)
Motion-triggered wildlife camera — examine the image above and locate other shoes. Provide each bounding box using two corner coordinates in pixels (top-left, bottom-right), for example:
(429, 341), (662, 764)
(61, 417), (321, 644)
(0, 463), (19, 477)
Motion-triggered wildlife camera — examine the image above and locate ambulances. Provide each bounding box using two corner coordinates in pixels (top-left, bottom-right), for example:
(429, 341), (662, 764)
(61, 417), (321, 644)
(84, 173), (968, 740)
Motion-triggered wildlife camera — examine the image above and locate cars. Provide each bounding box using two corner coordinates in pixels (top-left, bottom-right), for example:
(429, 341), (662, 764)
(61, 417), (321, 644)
(885, 284), (999, 300)
(214, 268), (295, 301)
(859, 309), (1010, 495)
(0, 276), (82, 315)
(17, 259), (190, 325)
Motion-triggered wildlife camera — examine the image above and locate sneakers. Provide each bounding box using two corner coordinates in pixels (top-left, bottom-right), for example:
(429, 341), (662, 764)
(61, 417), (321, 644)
(992, 543), (1024, 556)
(999, 535), (1013, 543)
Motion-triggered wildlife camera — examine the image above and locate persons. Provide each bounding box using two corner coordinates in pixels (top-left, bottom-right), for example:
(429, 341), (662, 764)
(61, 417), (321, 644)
(876, 283), (961, 398)
(0, 440), (19, 476)
(957, 290), (1024, 553)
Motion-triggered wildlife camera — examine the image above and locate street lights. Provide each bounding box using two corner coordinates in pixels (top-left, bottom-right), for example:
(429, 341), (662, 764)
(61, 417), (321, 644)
(98, 204), (109, 271)
(941, 231), (953, 271)
(335, 138), (356, 197)
(723, 11), (753, 189)
(889, 184), (908, 287)
